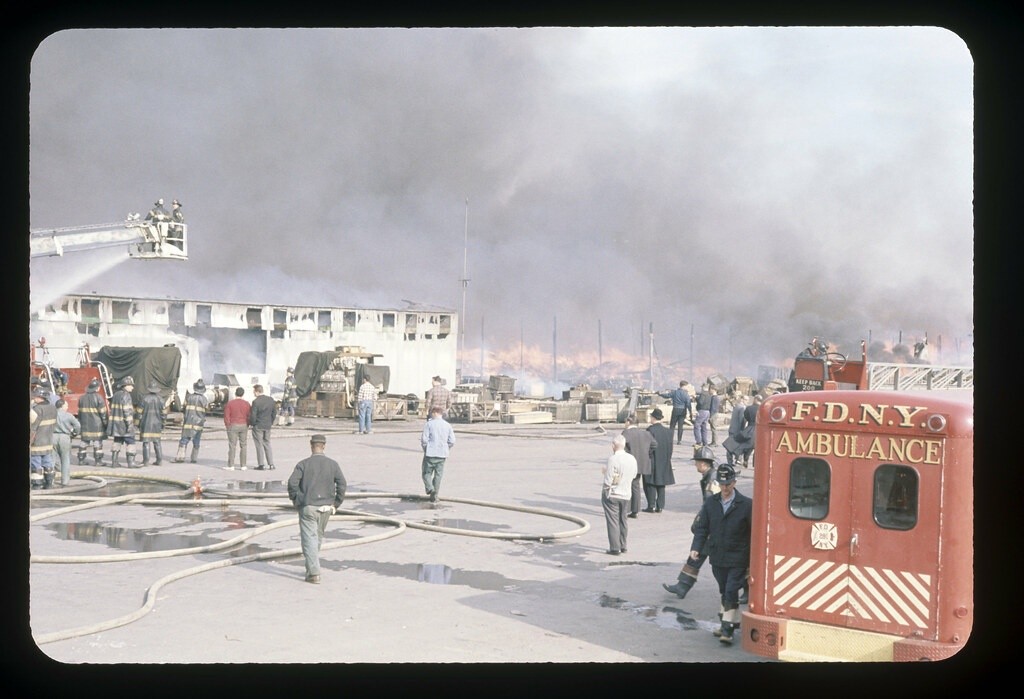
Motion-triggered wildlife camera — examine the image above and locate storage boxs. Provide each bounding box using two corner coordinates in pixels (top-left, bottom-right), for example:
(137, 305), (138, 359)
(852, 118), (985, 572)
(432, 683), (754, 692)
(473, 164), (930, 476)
(295, 346), (787, 426)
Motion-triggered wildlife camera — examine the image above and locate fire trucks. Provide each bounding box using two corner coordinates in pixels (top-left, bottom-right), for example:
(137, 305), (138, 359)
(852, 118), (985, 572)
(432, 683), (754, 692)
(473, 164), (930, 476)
(29, 211), (189, 415)
(742, 339), (973, 662)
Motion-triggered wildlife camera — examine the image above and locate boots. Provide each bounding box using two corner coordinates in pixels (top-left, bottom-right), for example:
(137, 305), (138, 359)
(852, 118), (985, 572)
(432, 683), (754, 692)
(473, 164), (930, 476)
(112, 451), (121, 467)
(78, 453), (92, 465)
(663, 582), (690, 599)
(141, 446), (163, 466)
(713, 613), (736, 644)
(126, 455), (145, 468)
(33, 474), (54, 489)
(95, 458), (107, 466)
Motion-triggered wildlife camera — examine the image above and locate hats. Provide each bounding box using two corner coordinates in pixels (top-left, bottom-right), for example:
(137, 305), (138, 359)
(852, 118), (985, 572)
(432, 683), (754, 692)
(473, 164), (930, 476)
(310, 435), (327, 443)
(650, 408), (664, 419)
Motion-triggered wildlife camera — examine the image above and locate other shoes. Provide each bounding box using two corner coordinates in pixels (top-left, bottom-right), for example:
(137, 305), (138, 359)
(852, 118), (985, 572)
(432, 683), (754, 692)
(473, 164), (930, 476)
(270, 465), (276, 469)
(628, 513), (638, 518)
(430, 491), (441, 503)
(169, 459), (185, 463)
(191, 460), (197, 463)
(354, 431), (373, 434)
(606, 548), (627, 555)
(223, 466), (235, 471)
(254, 465), (266, 470)
(241, 466), (248, 470)
(305, 571), (321, 584)
(642, 508), (663, 513)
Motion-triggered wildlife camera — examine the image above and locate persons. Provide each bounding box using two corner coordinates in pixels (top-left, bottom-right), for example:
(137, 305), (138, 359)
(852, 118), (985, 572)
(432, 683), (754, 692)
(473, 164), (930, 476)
(78, 376), (167, 468)
(169, 379), (209, 463)
(143, 198), (184, 252)
(601, 435), (638, 556)
(222, 385), (276, 470)
(621, 415), (658, 518)
(30, 387), (81, 490)
(288, 435), (346, 584)
(357, 375), (376, 434)
(658, 380), (763, 467)
(642, 409), (675, 513)
(420, 408), (454, 503)
(276, 368), (298, 425)
(424, 376), (452, 421)
(662, 446), (753, 604)
(690, 463), (753, 644)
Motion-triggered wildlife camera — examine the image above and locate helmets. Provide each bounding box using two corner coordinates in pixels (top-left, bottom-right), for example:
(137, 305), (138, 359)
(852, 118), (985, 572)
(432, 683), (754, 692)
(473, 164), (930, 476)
(34, 387), (50, 399)
(147, 381), (162, 394)
(118, 376), (136, 386)
(84, 380), (100, 393)
(193, 379), (207, 394)
(690, 447), (720, 463)
(716, 464), (740, 485)
